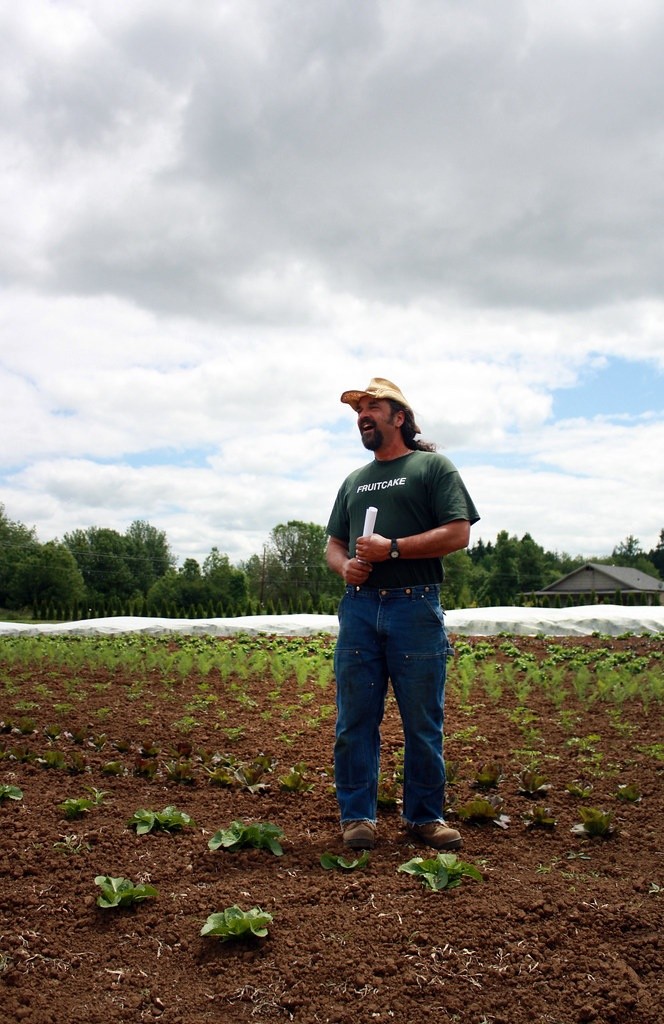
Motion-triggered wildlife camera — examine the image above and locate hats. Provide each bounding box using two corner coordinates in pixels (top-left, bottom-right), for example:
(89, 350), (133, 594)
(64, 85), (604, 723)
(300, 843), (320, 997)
(340, 377), (421, 435)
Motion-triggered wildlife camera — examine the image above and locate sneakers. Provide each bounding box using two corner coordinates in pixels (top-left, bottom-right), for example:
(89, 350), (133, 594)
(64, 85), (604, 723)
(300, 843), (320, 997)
(341, 820), (376, 849)
(407, 820), (465, 850)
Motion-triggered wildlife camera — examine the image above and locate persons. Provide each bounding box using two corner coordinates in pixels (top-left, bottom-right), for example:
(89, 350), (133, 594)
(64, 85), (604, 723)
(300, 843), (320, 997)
(326, 378), (481, 848)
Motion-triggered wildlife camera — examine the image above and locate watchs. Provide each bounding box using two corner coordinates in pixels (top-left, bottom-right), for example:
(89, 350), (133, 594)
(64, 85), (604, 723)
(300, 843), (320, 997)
(390, 538), (400, 560)
